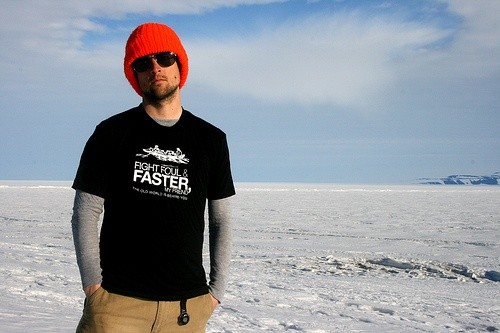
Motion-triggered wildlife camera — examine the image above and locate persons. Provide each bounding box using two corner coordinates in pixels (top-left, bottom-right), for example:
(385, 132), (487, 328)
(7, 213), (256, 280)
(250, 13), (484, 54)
(70, 22), (237, 333)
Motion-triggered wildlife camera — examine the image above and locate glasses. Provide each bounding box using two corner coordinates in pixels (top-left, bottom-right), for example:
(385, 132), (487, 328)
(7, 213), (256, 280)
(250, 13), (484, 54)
(132, 50), (178, 72)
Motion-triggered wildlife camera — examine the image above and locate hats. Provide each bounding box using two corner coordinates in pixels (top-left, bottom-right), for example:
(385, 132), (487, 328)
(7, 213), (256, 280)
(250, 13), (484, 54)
(124, 21), (189, 97)
